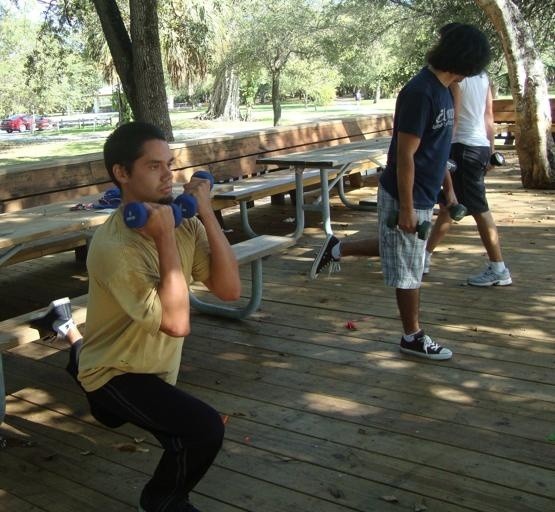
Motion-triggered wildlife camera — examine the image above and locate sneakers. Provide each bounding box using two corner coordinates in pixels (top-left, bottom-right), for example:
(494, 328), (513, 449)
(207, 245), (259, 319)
(423, 255), (431, 274)
(398, 329), (453, 361)
(471, 265), (513, 283)
(45, 296), (75, 335)
(309, 233), (342, 281)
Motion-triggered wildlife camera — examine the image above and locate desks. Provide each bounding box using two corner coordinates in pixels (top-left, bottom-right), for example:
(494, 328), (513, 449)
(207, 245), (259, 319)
(255, 136), (396, 245)
(0, 181), (296, 424)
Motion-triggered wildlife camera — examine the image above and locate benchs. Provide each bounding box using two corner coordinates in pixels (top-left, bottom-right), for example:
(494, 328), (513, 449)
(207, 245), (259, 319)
(491, 96), (555, 151)
(213, 153), (390, 241)
(0, 233), (295, 353)
(359, 195), (380, 211)
(0, 112), (398, 268)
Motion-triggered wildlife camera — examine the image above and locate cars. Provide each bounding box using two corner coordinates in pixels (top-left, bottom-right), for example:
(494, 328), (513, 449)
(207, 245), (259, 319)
(0, 113), (49, 134)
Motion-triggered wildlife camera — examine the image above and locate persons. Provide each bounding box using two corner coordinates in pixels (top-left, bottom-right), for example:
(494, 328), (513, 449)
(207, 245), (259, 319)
(423, 22), (512, 287)
(310, 24), (488, 360)
(31, 121), (242, 511)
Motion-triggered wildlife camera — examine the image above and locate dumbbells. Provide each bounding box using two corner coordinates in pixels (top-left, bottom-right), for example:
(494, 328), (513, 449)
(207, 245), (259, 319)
(386, 210), (432, 240)
(482, 152), (505, 176)
(174, 171), (214, 218)
(123, 202), (182, 228)
(447, 159), (457, 172)
(437, 189), (467, 221)
